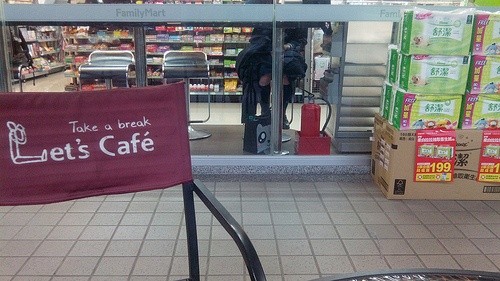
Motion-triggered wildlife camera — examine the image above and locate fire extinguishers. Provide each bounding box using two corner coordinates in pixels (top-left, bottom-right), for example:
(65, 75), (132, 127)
(298, 87), (332, 136)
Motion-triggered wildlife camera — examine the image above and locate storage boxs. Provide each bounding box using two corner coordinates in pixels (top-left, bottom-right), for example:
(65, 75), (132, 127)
(370, 115), (500, 202)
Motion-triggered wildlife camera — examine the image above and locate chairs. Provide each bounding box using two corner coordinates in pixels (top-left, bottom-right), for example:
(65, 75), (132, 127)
(8, 60), (36, 93)
(160, 51), (212, 140)
(78, 49), (136, 89)
